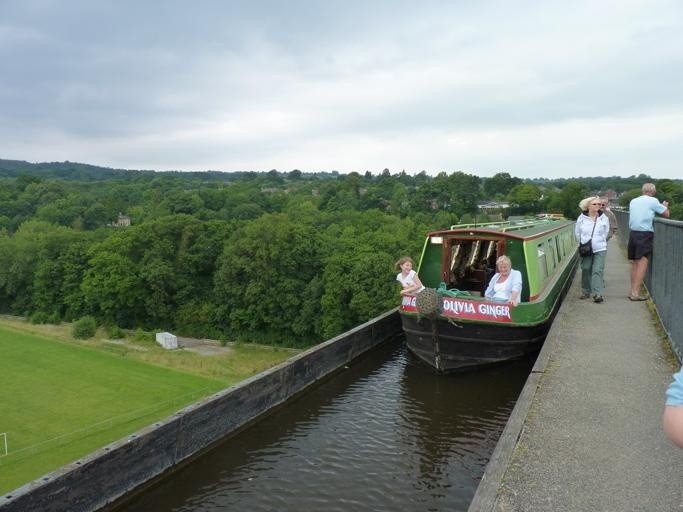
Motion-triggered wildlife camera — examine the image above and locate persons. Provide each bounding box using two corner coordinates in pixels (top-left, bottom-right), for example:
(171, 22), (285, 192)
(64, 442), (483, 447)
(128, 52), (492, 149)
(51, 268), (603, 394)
(626, 182), (670, 301)
(483, 254), (523, 307)
(394, 256), (426, 294)
(574, 196), (609, 302)
(595, 196), (618, 289)
(662, 366), (683, 450)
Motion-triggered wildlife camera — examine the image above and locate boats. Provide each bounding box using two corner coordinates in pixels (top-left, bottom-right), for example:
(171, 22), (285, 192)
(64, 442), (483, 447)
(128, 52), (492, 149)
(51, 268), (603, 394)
(394, 209), (582, 380)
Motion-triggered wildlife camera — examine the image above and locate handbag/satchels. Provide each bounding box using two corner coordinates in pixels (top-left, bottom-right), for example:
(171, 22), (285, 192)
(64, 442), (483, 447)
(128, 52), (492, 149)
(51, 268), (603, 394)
(578, 238), (593, 258)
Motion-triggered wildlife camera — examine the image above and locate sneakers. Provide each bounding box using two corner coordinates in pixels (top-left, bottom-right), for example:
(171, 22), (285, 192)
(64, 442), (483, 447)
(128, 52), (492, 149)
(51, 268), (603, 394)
(629, 295), (647, 301)
(594, 294), (603, 302)
(579, 293), (591, 300)
